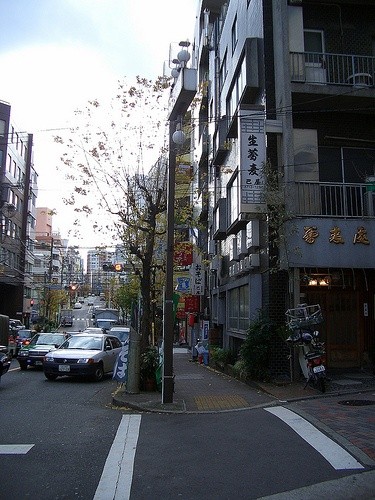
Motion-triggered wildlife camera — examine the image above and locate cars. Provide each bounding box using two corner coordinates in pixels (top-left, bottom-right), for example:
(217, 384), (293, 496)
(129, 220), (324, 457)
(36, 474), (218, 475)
(42, 334), (124, 382)
(17, 332), (72, 370)
(0, 292), (131, 376)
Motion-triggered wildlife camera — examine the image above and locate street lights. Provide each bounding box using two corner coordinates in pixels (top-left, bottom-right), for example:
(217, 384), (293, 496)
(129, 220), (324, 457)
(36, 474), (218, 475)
(160, 49), (200, 403)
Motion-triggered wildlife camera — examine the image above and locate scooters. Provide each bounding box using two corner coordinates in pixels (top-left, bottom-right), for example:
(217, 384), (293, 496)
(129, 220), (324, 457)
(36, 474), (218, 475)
(295, 329), (328, 393)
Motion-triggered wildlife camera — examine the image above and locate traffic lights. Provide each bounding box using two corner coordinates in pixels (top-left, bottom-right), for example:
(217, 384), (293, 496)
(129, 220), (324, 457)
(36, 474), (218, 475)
(101, 263), (123, 273)
